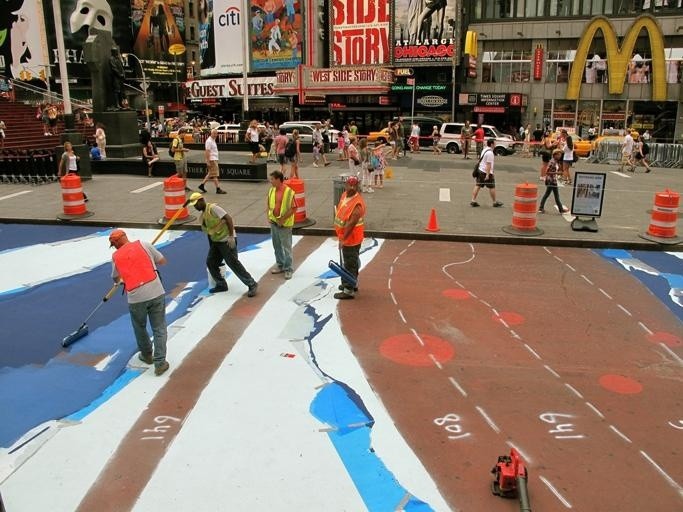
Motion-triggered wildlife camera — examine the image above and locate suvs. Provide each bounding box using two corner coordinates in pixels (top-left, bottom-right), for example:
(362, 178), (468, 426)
(389, 117), (450, 150)
(277, 121), (344, 150)
(435, 123), (516, 157)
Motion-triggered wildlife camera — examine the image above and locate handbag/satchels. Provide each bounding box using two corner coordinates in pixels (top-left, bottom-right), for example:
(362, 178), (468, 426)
(366, 149), (373, 172)
(73, 151), (80, 170)
(472, 149), (493, 178)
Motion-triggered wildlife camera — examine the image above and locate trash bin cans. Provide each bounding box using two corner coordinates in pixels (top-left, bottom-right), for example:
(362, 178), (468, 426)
(333, 174), (362, 226)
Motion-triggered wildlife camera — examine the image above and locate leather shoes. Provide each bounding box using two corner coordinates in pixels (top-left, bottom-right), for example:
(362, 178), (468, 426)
(139, 352), (152, 364)
(210, 285), (229, 293)
(199, 185), (208, 192)
(155, 360), (168, 375)
(185, 187), (192, 190)
(272, 268), (285, 274)
(338, 285), (359, 292)
(324, 162), (331, 167)
(248, 282), (257, 296)
(334, 292), (353, 299)
(493, 201), (503, 207)
(285, 270), (292, 278)
(216, 189), (226, 194)
(471, 202), (480, 206)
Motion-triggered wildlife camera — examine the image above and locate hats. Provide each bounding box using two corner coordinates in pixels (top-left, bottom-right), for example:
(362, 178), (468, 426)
(189, 193), (204, 205)
(347, 176), (359, 185)
(109, 230), (127, 246)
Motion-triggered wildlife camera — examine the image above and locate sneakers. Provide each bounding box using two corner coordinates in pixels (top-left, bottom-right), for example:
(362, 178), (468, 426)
(538, 209), (544, 212)
(560, 208), (569, 213)
(645, 168), (651, 173)
(44, 133), (48, 135)
(316, 165), (318, 168)
(313, 163), (315, 166)
(48, 133), (53, 136)
(628, 167), (635, 172)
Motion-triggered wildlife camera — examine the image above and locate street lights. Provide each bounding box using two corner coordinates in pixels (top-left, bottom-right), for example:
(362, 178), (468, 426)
(122, 53), (151, 133)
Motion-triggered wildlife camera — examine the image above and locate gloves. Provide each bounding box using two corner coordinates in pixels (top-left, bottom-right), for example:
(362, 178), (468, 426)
(227, 237), (237, 249)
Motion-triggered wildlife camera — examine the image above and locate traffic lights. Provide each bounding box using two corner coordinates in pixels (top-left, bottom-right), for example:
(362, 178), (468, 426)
(39, 69), (44, 78)
(26, 71), (32, 81)
(19, 71), (25, 81)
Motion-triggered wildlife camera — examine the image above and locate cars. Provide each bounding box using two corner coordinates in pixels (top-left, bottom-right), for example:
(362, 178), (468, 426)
(211, 124), (249, 143)
(542, 127), (591, 158)
(169, 127), (209, 144)
(592, 129), (641, 160)
(368, 128), (394, 146)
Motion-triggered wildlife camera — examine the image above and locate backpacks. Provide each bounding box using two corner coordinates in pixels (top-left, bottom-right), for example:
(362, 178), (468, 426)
(642, 142), (649, 154)
(284, 139), (297, 158)
(168, 138), (179, 157)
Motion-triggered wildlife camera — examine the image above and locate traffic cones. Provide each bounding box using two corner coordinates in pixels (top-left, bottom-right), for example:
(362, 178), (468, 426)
(157, 176), (196, 225)
(56, 173), (95, 220)
(282, 179), (316, 229)
(425, 207), (440, 234)
(638, 189), (683, 244)
(501, 182), (544, 236)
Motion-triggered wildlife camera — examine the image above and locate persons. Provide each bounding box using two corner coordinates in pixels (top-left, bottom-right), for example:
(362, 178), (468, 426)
(537, 149), (569, 213)
(143, 139), (160, 177)
(0, 120), (6, 147)
(342, 124), (351, 161)
(588, 123), (596, 140)
(320, 119), (334, 142)
(522, 123), (531, 152)
(429, 125), (442, 155)
(466, 124), (484, 159)
(169, 127), (191, 191)
(388, 118), (421, 160)
(283, 0), (296, 25)
(334, 175), (366, 301)
(558, 129), (571, 180)
(359, 138), (375, 193)
(540, 132), (559, 179)
(75, 108), (95, 127)
(349, 121), (358, 135)
(615, 130), (637, 172)
(312, 142), (321, 168)
(519, 124), (524, 139)
(269, 18), (282, 56)
(92, 122), (107, 159)
(35, 104), (44, 120)
(263, 121), (273, 153)
(48, 104), (58, 135)
(144, 116), (235, 139)
(245, 119), (265, 162)
(285, 128), (303, 178)
(90, 142), (101, 160)
(57, 141), (88, 202)
(275, 128), (288, 176)
(268, 123), (280, 161)
(289, 30), (298, 61)
(470, 138), (504, 208)
(313, 123), (331, 167)
(371, 142), (385, 188)
(267, 170), (298, 280)
(40, 103), (52, 136)
(460, 120), (472, 159)
(198, 129), (227, 194)
(252, 10), (264, 42)
(348, 137), (360, 177)
(556, 137), (562, 150)
(558, 136), (573, 185)
(627, 136), (651, 173)
(109, 229), (170, 377)
(532, 123), (544, 158)
(337, 132), (345, 161)
(631, 128), (639, 140)
(188, 192), (259, 298)
(642, 129), (652, 142)
